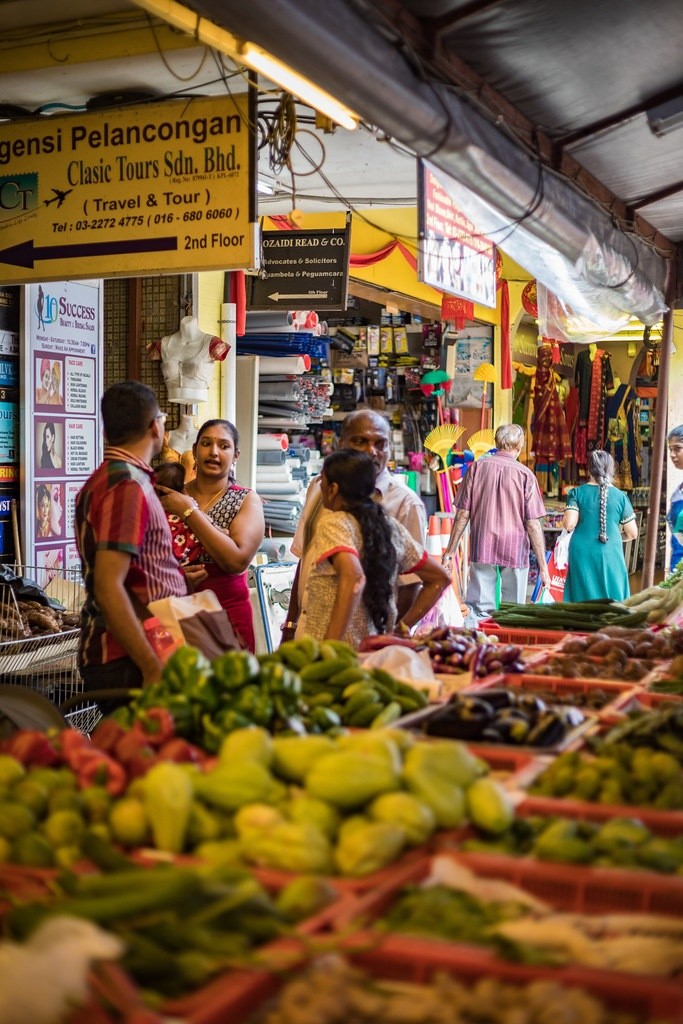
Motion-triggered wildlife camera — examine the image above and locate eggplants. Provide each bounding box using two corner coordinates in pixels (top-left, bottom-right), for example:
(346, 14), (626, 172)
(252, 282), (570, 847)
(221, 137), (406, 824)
(354, 623), (521, 678)
(424, 687), (577, 747)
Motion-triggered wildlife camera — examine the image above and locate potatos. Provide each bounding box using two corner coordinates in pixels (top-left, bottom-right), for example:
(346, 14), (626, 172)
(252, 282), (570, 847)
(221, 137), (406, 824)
(0, 598), (83, 655)
(560, 624), (683, 660)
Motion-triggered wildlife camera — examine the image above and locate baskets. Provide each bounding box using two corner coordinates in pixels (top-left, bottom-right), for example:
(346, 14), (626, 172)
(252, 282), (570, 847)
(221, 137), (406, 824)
(1, 612), (682, 1024)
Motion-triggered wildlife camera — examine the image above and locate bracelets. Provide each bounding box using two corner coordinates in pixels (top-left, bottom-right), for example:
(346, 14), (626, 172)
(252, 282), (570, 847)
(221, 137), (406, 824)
(180, 505), (201, 520)
(393, 619), (412, 637)
(440, 554), (454, 562)
(280, 620), (300, 634)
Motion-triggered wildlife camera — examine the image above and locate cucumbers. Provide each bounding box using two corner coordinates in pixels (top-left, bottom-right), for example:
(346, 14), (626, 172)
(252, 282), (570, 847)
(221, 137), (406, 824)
(253, 634), (431, 732)
(492, 598), (648, 632)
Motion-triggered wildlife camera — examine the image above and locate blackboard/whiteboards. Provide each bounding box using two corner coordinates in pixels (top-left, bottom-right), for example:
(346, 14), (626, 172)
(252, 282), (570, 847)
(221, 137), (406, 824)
(247, 211), (352, 312)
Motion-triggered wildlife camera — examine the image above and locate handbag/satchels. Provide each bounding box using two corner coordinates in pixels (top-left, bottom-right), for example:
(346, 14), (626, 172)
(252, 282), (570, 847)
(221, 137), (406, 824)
(410, 568), (465, 637)
(531, 551), (569, 603)
(147, 589), (241, 662)
(553, 526), (574, 570)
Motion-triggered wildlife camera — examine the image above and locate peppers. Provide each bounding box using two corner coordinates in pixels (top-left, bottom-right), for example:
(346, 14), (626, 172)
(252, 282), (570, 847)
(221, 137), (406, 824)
(0, 643), (344, 799)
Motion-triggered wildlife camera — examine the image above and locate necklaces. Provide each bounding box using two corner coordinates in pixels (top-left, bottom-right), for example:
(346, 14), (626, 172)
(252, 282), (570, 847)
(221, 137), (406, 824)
(186, 478), (230, 511)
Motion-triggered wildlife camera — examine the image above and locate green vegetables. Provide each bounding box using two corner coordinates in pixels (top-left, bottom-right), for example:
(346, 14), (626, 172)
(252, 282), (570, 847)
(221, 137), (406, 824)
(0, 831), (303, 1004)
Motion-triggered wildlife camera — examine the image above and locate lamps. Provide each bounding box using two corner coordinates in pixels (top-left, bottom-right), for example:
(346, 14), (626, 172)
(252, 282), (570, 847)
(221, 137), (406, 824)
(235, 37), (360, 131)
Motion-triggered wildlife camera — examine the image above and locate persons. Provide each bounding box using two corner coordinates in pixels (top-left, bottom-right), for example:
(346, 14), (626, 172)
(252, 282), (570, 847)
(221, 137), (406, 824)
(433, 422), (550, 629)
(561, 449), (640, 605)
(71, 382), (267, 723)
(659, 423), (683, 579)
(276, 406), (450, 651)
(145, 316), (231, 483)
(530, 343), (638, 498)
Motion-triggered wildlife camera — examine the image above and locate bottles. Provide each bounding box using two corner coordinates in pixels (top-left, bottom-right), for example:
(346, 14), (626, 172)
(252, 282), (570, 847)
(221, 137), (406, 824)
(384, 366), (400, 404)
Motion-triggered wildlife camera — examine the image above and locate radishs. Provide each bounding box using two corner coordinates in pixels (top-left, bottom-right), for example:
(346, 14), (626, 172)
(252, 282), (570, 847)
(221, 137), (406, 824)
(622, 577), (683, 623)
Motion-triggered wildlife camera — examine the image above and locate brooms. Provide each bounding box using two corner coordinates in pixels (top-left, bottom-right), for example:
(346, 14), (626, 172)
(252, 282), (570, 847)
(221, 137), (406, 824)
(466, 428), (497, 461)
(422, 422), (469, 514)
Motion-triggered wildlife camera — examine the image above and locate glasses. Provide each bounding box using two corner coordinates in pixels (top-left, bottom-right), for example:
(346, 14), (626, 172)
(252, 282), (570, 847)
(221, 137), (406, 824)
(149, 412), (168, 428)
